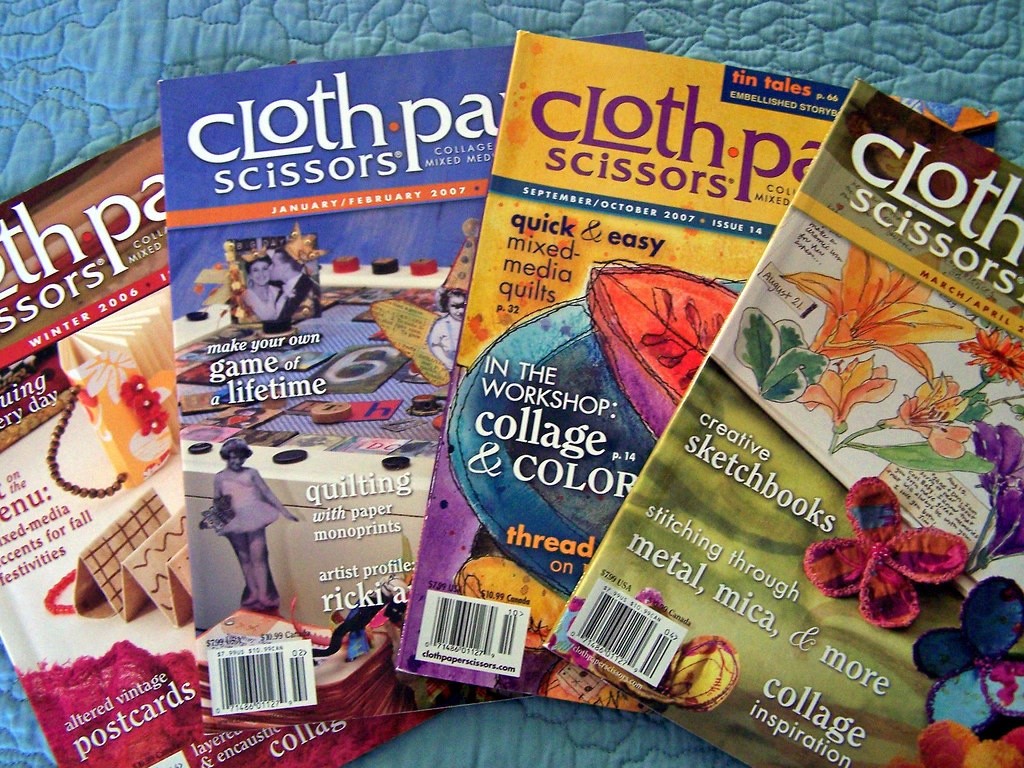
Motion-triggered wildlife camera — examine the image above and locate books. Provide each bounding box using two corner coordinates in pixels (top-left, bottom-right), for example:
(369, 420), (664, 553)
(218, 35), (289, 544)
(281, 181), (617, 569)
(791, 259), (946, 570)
(0, 29), (1024, 768)
